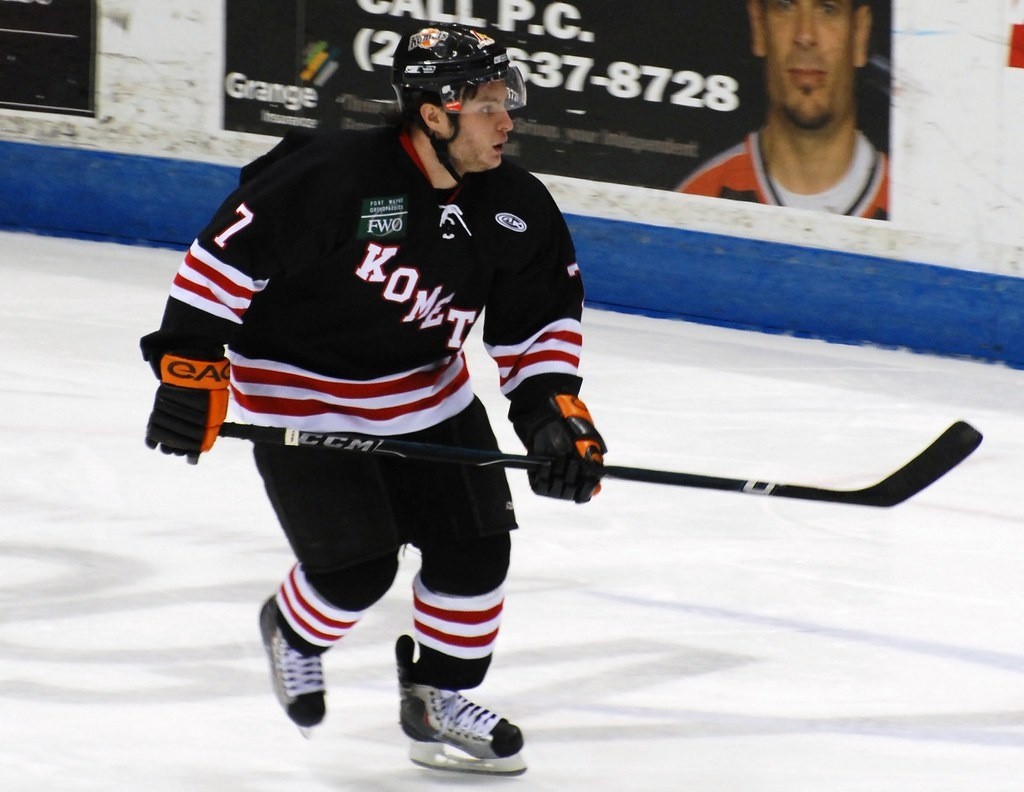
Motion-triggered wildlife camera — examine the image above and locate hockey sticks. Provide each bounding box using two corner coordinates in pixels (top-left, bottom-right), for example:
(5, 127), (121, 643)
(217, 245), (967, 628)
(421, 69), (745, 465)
(215, 421), (984, 506)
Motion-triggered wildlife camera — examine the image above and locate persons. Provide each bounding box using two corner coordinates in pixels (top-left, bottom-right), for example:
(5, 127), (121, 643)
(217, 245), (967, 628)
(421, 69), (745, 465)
(144, 24), (606, 778)
(676, 0), (891, 220)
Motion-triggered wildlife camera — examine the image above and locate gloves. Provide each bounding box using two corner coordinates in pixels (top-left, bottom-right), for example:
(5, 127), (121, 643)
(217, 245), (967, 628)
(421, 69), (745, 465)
(512, 395), (606, 504)
(145, 354), (231, 465)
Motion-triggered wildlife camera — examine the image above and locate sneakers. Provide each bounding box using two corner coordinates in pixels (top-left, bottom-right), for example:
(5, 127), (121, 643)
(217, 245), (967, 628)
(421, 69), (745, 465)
(259, 597), (325, 740)
(395, 634), (526, 777)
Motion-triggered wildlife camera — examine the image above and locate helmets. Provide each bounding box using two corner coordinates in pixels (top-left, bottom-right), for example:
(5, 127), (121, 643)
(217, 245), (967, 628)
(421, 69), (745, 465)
(390, 22), (510, 114)
